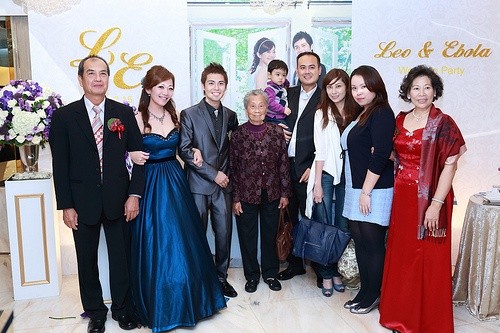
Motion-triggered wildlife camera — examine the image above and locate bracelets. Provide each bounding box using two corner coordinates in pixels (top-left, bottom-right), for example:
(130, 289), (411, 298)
(361, 191), (371, 197)
(431, 198), (444, 204)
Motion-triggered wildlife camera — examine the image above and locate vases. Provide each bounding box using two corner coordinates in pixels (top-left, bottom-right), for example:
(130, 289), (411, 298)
(13, 143), (51, 180)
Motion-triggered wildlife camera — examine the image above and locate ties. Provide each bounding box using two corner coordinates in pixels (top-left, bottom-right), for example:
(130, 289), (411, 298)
(214, 109), (219, 117)
(92, 106), (104, 178)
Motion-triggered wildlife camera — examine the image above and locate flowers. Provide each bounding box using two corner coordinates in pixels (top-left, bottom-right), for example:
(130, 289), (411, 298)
(108, 118), (125, 140)
(0, 78), (65, 149)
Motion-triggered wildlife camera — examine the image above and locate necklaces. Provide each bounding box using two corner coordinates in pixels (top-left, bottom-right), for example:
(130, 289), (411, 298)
(148, 108), (165, 125)
(413, 109), (429, 121)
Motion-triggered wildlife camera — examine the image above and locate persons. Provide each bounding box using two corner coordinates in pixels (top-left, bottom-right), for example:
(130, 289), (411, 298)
(248, 37), (275, 90)
(48, 54), (145, 333)
(178, 62), (239, 297)
(292, 31), (326, 89)
(127, 65), (203, 308)
(263, 59), (291, 137)
(340, 65), (396, 314)
(229, 88), (290, 293)
(277, 51), (324, 289)
(312, 69), (360, 297)
(371, 64), (459, 333)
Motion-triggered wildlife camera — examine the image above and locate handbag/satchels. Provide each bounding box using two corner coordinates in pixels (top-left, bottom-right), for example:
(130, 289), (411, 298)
(275, 207), (293, 262)
(336, 238), (362, 285)
(293, 217), (350, 265)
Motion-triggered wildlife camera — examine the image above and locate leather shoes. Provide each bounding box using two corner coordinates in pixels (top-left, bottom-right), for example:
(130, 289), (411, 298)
(87, 316), (107, 333)
(112, 313), (138, 330)
(221, 281), (237, 297)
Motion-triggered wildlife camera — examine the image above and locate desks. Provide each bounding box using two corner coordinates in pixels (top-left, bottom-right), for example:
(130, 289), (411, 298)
(451, 190), (500, 321)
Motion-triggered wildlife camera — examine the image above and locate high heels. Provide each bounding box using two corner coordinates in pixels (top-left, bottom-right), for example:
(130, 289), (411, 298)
(344, 293), (381, 314)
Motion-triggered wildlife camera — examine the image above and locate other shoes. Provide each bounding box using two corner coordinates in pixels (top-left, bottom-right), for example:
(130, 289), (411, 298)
(334, 283), (345, 292)
(245, 280), (259, 292)
(264, 279), (282, 291)
(317, 277), (324, 288)
(276, 267), (306, 280)
(322, 285), (334, 296)
